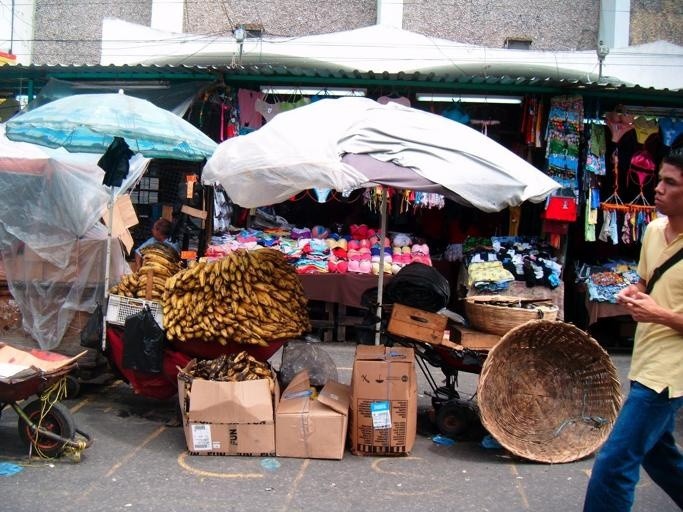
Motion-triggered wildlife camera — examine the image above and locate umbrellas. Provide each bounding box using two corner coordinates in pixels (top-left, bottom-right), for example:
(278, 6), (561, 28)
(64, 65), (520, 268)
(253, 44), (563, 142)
(5, 87), (220, 352)
(202, 96), (566, 345)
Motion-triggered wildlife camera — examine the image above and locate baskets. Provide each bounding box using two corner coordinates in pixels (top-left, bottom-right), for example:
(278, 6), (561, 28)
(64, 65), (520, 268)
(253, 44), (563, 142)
(465, 300), (623, 463)
(106, 294), (165, 334)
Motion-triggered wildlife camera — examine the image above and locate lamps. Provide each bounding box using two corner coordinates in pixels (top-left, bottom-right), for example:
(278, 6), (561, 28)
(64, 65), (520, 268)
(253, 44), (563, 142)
(235, 24), (246, 66)
(69, 78), (171, 91)
(259, 84), (369, 97)
(415, 91), (524, 105)
(596, 39), (610, 82)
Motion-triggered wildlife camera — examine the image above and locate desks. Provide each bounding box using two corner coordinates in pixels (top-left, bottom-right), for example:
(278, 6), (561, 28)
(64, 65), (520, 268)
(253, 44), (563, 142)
(295, 271), (391, 343)
(583, 286), (634, 325)
(460, 264), (565, 320)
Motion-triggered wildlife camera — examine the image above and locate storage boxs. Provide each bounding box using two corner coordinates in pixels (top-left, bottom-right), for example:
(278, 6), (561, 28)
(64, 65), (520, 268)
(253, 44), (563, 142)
(174, 343), (419, 461)
(386, 303), (449, 345)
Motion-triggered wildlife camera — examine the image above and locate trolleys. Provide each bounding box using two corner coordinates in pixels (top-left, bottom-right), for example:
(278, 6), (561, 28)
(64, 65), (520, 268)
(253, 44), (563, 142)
(351, 305), (490, 437)
(1, 342), (97, 457)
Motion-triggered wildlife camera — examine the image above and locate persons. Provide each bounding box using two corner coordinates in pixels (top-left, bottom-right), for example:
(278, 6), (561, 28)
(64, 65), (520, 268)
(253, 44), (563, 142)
(135, 219), (180, 271)
(582, 149), (683, 512)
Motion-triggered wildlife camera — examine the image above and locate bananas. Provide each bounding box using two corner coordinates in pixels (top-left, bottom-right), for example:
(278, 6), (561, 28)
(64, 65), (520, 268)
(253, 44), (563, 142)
(110, 241), (314, 418)
